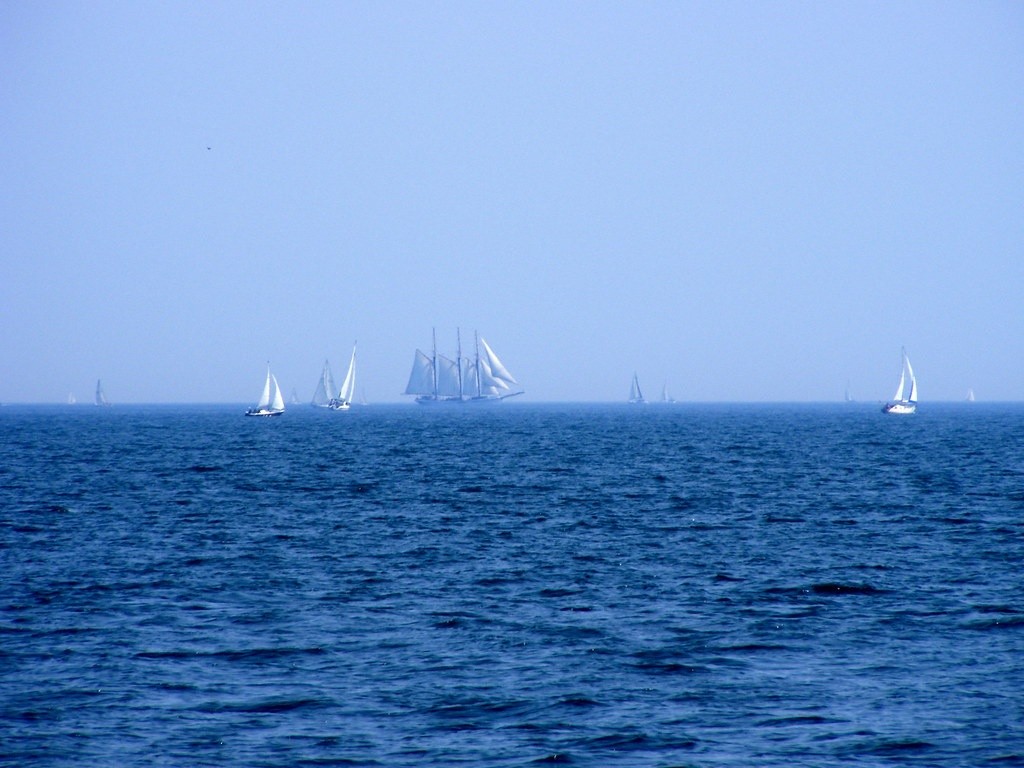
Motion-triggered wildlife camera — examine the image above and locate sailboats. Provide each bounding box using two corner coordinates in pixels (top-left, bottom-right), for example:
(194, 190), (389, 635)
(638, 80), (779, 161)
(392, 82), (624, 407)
(328, 339), (359, 411)
(243, 363), (286, 417)
(308, 357), (335, 407)
(882, 345), (919, 415)
(627, 373), (677, 406)
(403, 326), (527, 406)
